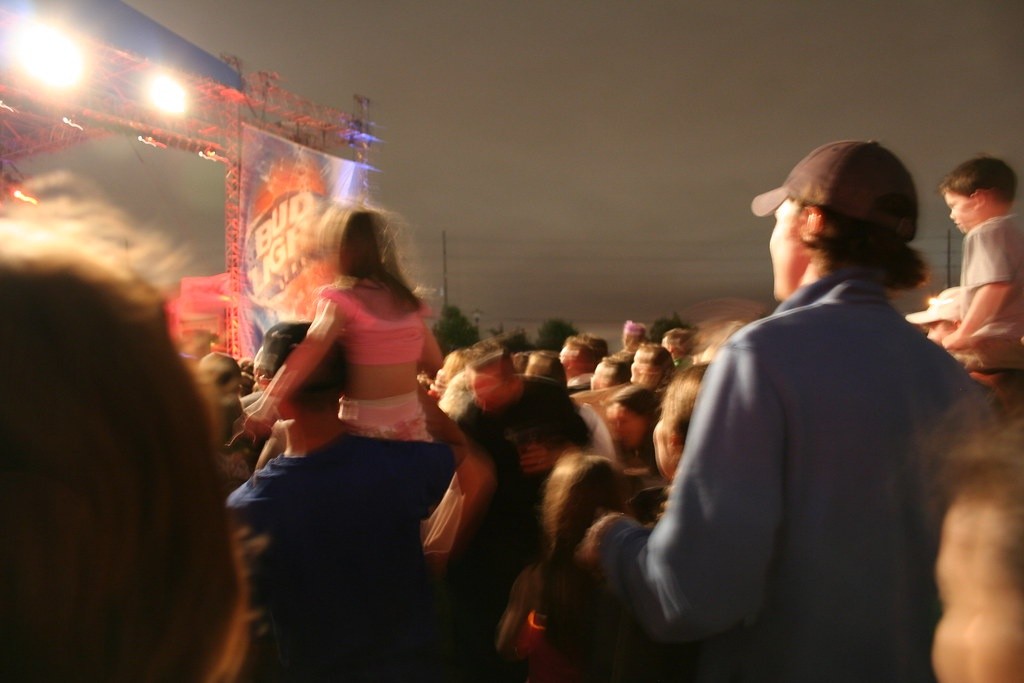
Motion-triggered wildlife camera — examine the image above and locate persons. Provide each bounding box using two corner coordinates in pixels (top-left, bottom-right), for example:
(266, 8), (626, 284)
(182, 202), (753, 683)
(900, 148), (1024, 683)
(0, 204), (247, 683)
(583, 141), (995, 683)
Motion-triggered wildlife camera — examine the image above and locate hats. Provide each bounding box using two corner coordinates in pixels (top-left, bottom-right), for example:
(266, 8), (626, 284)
(905, 288), (964, 330)
(750, 140), (918, 243)
